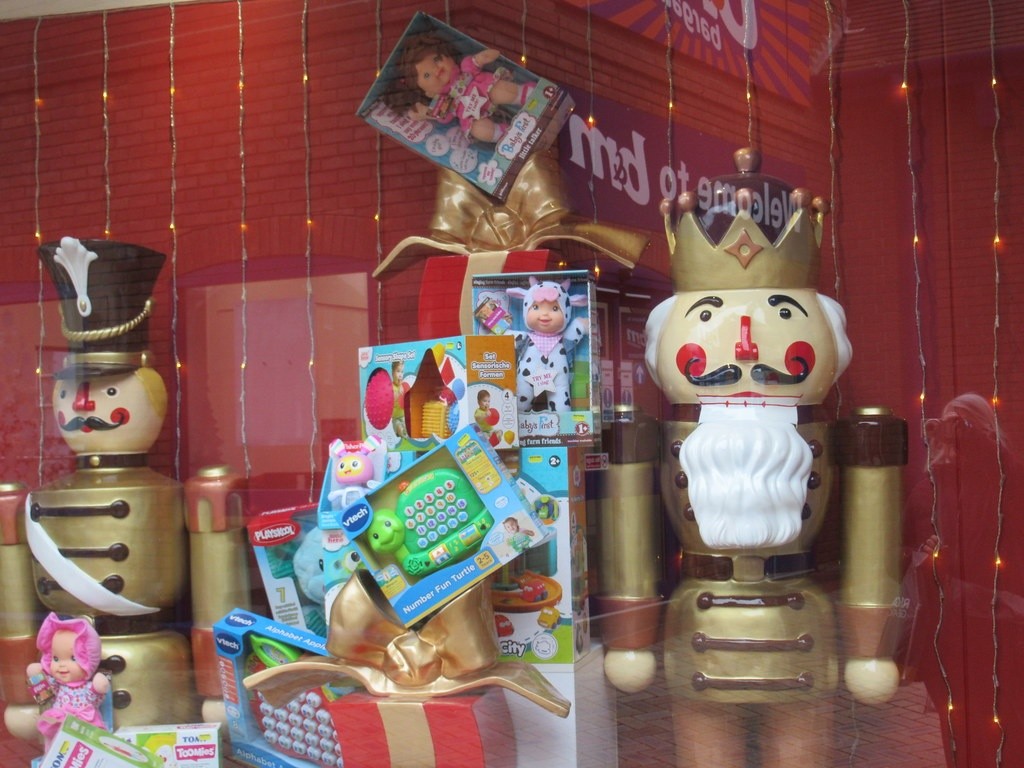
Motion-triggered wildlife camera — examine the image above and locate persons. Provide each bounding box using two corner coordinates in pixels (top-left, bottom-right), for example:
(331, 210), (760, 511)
(387, 32), (540, 146)
(0, 236), (254, 734)
(475, 390), (494, 439)
(391, 360), (407, 439)
(502, 516), (535, 552)
(499, 277), (587, 411)
(601, 146), (909, 768)
(904, 393), (1024, 683)
(29, 612), (108, 757)
